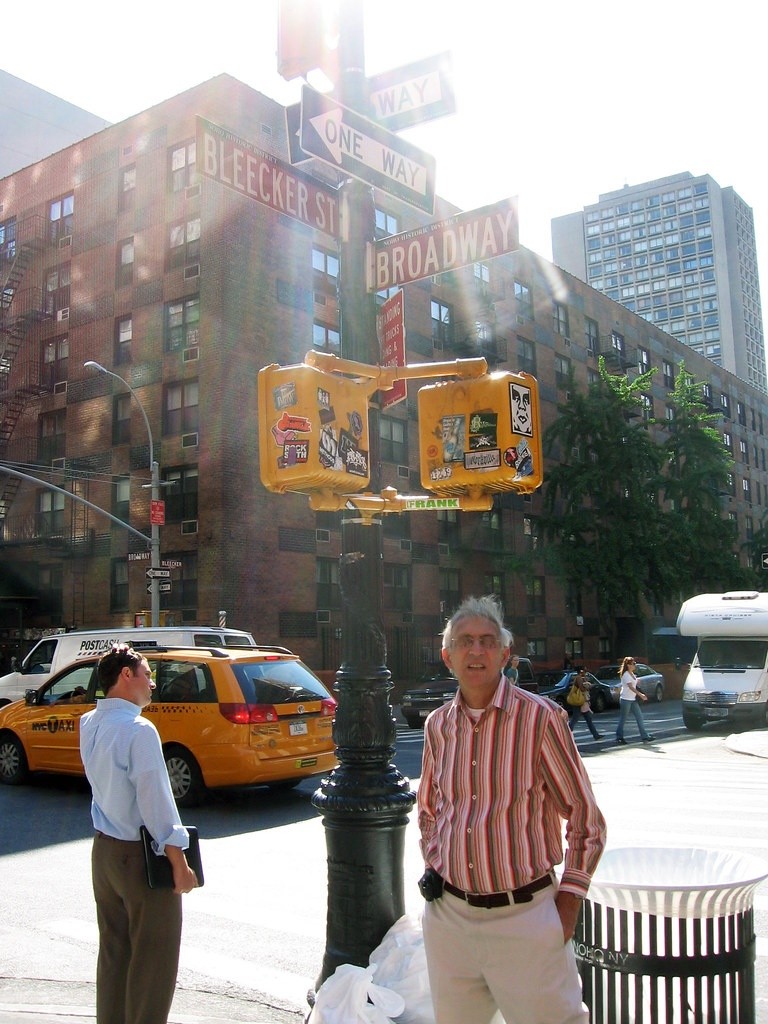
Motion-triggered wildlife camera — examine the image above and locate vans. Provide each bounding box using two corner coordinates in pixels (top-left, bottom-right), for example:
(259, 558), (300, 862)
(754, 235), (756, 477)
(0, 626), (259, 709)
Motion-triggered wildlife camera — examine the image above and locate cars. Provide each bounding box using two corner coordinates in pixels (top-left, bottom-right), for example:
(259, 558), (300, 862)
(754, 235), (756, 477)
(531, 671), (612, 716)
(592, 663), (665, 705)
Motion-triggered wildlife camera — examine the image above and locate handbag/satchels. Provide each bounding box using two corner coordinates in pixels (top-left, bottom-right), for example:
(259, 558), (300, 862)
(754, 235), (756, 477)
(566, 677), (585, 705)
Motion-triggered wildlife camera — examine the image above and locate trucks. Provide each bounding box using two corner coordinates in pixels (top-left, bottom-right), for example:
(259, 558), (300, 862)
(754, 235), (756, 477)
(675, 590), (768, 733)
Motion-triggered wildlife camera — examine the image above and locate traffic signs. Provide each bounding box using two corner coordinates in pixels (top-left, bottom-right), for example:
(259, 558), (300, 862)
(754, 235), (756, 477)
(144, 567), (171, 579)
(147, 582), (173, 595)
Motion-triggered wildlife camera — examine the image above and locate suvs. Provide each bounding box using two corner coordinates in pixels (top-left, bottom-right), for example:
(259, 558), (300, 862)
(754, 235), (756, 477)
(0, 644), (340, 807)
(401, 654), (539, 730)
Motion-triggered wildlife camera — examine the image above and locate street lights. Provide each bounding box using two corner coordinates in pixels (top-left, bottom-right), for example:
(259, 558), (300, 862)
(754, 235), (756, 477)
(82, 360), (161, 630)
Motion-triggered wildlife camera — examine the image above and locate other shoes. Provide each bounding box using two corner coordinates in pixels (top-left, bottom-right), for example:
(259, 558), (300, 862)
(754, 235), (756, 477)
(615, 738), (628, 745)
(595, 734), (606, 741)
(642, 737), (654, 741)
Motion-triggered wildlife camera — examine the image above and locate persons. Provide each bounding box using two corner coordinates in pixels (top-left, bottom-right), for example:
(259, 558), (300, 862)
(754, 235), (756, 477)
(78, 643), (204, 1024)
(49, 687), (88, 707)
(505, 655), (520, 687)
(615, 657), (656, 745)
(568, 668), (606, 741)
(417, 598), (608, 1024)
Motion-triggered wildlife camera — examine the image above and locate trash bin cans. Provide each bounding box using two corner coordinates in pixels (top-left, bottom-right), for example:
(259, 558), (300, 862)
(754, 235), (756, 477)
(555, 847), (768, 1024)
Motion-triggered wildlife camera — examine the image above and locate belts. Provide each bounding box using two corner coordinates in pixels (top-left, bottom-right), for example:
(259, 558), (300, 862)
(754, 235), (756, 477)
(443, 868), (556, 910)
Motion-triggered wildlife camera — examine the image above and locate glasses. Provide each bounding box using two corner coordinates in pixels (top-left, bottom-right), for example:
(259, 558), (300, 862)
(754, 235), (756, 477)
(633, 661), (636, 665)
(451, 635), (501, 649)
